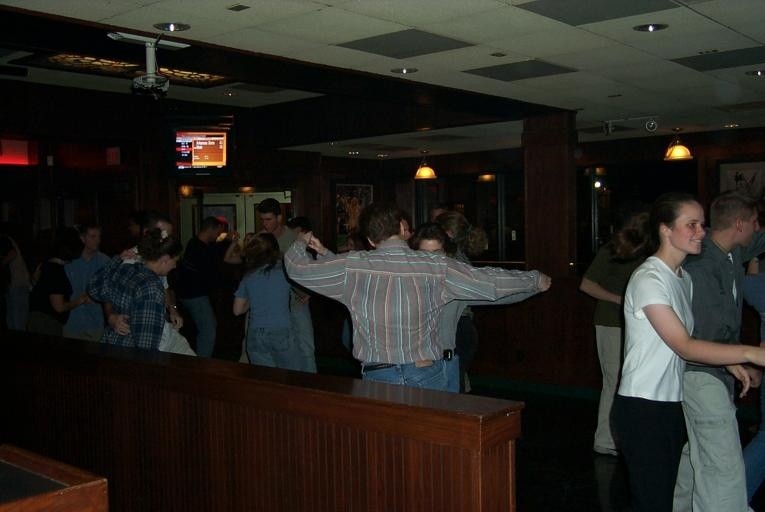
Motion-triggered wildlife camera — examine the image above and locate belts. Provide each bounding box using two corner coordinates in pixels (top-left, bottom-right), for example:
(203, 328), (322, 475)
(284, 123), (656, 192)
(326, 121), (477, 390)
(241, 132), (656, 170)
(361, 363), (396, 373)
(444, 347), (458, 361)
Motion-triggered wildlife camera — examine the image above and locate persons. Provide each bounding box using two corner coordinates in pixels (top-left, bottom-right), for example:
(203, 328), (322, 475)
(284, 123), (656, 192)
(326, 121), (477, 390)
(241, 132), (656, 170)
(576, 212), (658, 456)
(742, 258), (763, 505)
(1, 197), (553, 397)
(673, 194), (757, 510)
(607, 197), (765, 511)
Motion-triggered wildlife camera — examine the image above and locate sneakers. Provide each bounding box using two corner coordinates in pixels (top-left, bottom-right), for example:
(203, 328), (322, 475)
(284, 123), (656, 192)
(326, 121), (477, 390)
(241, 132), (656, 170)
(591, 442), (621, 457)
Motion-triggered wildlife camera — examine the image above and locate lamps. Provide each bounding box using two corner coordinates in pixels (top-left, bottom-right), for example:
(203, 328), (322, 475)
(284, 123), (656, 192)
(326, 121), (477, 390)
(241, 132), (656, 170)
(664, 127), (693, 161)
(414, 149), (437, 179)
(131, 31), (171, 96)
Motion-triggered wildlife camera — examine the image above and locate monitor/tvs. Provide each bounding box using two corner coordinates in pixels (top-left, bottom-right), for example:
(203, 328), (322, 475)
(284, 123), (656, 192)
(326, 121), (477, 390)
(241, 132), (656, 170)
(173, 126), (232, 176)
